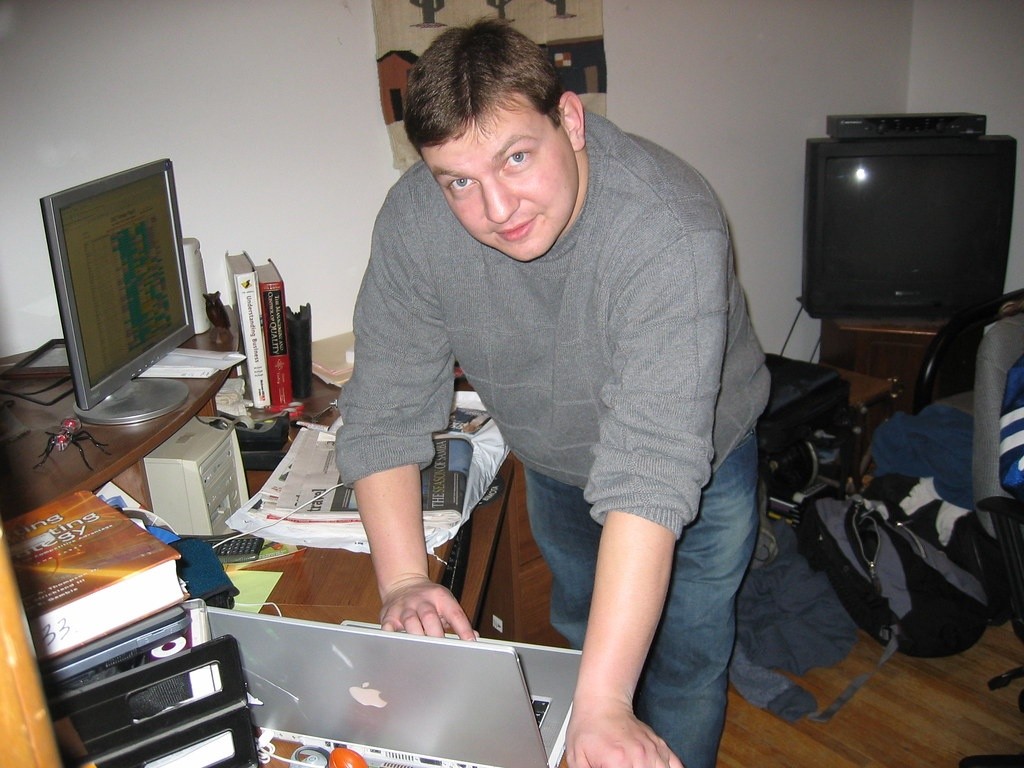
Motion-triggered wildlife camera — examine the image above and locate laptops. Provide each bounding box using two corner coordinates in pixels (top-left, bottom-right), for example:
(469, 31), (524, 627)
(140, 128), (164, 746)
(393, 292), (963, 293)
(203, 605), (584, 768)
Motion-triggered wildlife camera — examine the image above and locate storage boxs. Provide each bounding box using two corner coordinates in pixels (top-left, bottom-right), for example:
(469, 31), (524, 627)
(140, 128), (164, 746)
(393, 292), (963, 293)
(143, 416), (250, 535)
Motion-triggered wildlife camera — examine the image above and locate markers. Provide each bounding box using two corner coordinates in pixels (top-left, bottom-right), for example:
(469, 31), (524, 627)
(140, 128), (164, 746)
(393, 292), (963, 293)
(289, 420), (331, 432)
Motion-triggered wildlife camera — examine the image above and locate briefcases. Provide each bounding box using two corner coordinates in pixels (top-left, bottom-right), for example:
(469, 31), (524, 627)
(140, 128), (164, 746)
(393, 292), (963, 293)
(752, 354), (839, 456)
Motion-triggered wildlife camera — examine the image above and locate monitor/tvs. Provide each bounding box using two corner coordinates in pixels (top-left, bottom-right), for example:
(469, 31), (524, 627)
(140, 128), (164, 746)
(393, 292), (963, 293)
(799, 131), (1019, 318)
(40, 158), (195, 427)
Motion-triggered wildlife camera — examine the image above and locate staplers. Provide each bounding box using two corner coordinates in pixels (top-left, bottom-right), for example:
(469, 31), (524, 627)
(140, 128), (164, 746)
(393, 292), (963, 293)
(263, 400), (304, 419)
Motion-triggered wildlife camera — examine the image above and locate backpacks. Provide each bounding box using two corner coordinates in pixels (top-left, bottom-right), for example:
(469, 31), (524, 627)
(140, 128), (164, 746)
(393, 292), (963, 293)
(795, 484), (989, 658)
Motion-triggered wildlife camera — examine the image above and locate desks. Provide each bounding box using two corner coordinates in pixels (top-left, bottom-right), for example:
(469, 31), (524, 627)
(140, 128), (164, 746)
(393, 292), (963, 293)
(818, 316), (952, 427)
(0, 305), (517, 649)
(813, 361), (893, 448)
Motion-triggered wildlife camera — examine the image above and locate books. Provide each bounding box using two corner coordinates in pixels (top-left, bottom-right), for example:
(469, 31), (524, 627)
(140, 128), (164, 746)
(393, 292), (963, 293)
(1, 488), (192, 665)
(224, 249), (313, 411)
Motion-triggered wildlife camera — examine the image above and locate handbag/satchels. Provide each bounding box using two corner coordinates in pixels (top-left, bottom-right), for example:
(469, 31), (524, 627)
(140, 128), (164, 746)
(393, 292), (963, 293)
(755, 408), (871, 513)
(747, 477), (778, 570)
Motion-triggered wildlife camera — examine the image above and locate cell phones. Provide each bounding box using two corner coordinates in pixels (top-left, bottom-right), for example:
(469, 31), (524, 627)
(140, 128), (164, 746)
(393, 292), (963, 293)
(200, 536), (265, 565)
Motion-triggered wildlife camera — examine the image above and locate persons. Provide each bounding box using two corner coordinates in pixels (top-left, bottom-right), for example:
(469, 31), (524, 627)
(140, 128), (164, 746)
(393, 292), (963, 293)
(997, 357), (1024, 712)
(332, 19), (778, 768)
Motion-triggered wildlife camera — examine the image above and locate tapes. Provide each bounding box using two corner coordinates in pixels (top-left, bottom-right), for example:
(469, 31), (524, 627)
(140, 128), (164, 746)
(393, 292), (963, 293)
(233, 415), (255, 430)
